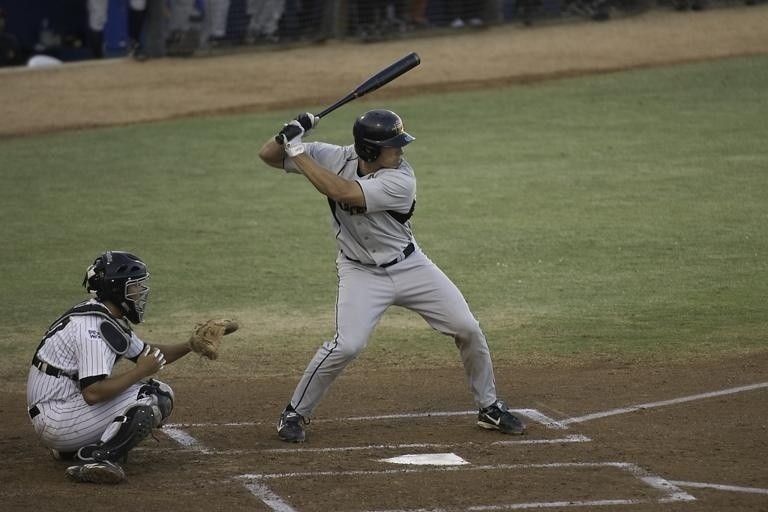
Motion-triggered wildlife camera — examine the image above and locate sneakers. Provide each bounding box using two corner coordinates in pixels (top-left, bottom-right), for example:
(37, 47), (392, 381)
(66, 459), (124, 485)
(277, 408), (310, 442)
(477, 401), (525, 434)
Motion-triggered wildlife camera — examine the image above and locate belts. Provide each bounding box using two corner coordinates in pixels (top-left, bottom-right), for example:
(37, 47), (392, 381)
(375, 243), (414, 268)
(29, 405), (40, 418)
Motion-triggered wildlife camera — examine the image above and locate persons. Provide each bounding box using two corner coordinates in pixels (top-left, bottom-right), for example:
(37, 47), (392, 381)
(256, 108), (525, 443)
(1, 1), (705, 68)
(26, 251), (238, 482)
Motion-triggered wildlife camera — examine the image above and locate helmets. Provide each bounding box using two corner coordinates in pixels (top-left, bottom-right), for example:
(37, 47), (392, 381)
(84, 249), (150, 325)
(352, 110), (416, 163)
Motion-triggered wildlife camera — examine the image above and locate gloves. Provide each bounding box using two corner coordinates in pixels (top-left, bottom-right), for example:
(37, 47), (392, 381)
(276, 112), (319, 158)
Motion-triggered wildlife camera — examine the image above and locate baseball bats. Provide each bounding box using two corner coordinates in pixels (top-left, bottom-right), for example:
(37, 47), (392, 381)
(276, 53), (420, 144)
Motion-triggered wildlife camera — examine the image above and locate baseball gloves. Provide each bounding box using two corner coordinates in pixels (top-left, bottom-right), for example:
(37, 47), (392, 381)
(191, 318), (239, 360)
